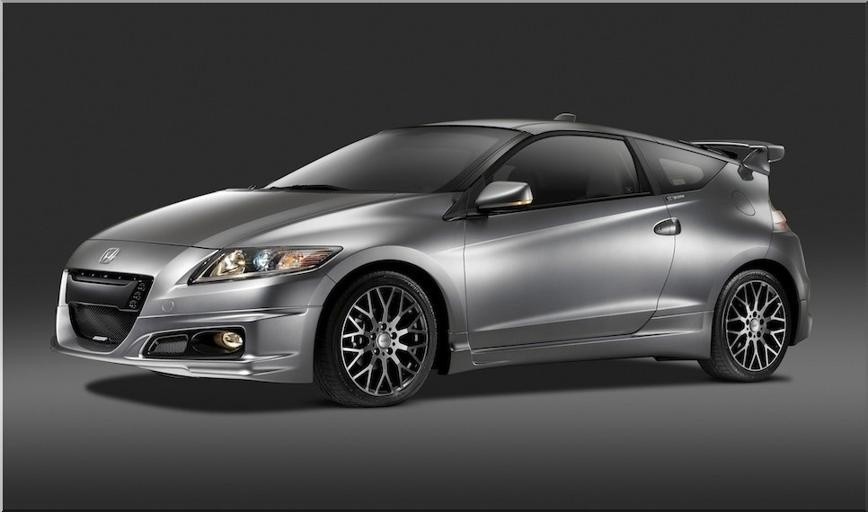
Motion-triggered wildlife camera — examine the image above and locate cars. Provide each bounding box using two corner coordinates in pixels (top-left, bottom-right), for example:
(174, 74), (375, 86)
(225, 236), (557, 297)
(51, 111), (814, 410)
